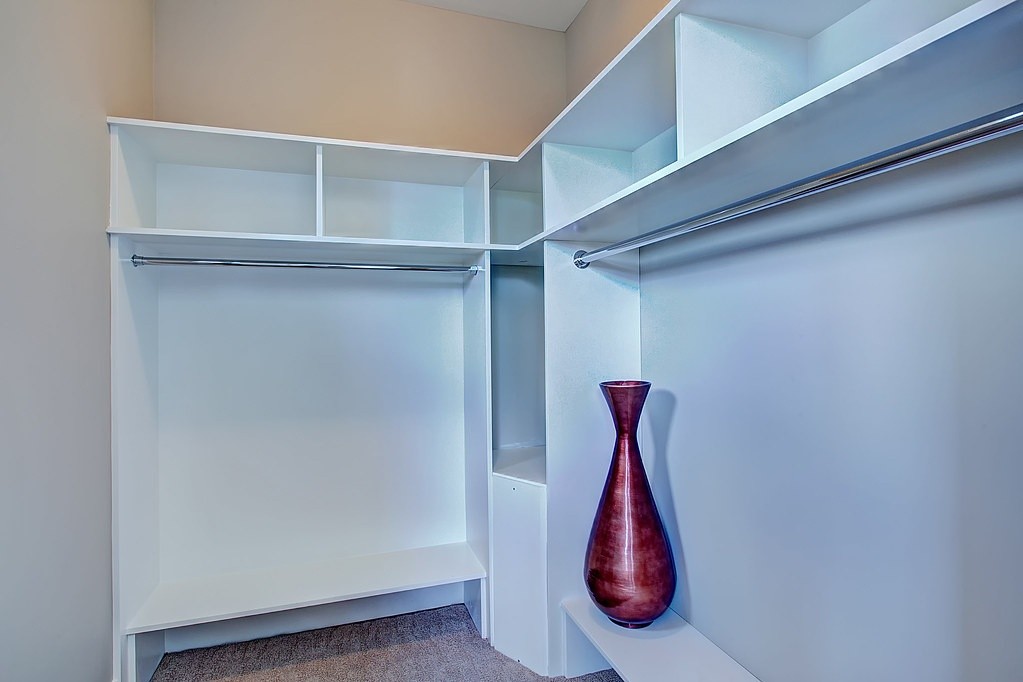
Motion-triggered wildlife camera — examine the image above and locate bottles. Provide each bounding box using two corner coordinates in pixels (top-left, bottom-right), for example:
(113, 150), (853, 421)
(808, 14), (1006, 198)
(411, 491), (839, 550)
(583, 379), (677, 629)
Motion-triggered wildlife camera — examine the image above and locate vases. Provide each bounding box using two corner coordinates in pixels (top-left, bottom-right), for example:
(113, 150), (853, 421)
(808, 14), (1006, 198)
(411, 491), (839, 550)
(583, 381), (677, 629)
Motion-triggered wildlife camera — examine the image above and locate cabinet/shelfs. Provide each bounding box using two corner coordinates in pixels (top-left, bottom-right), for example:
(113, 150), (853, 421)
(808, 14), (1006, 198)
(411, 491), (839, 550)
(106, 1), (1022, 681)
